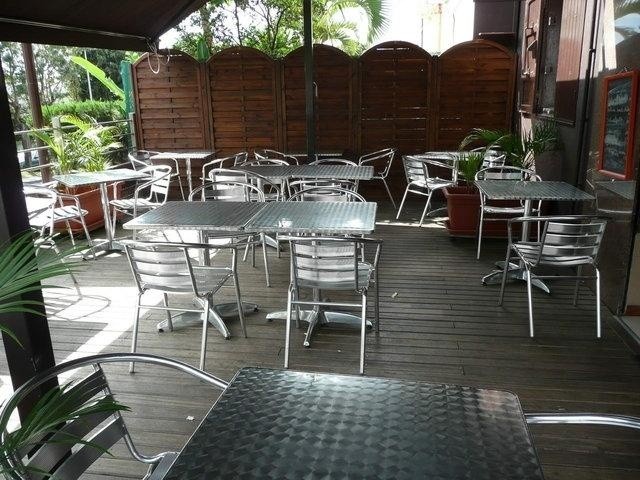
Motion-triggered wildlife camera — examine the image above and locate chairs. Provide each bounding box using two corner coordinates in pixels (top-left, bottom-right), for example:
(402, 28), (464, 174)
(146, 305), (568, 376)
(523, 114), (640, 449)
(397, 149), (509, 227)
(475, 165), (543, 260)
(118, 238), (248, 375)
(497, 213), (610, 339)
(0, 350), (230, 480)
(273, 232), (386, 377)
(22, 145), (398, 286)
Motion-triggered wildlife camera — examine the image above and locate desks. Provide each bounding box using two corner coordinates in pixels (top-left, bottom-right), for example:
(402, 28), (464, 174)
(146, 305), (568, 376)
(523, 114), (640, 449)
(245, 201), (378, 348)
(121, 199), (270, 342)
(472, 177), (596, 294)
(161, 362), (546, 480)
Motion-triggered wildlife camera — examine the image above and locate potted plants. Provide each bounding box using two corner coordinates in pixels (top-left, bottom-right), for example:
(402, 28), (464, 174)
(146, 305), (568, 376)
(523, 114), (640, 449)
(22, 110), (133, 232)
(437, 113), (566, 236)
